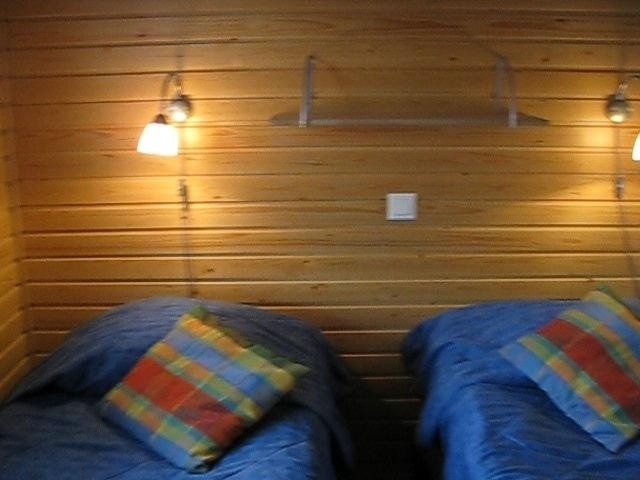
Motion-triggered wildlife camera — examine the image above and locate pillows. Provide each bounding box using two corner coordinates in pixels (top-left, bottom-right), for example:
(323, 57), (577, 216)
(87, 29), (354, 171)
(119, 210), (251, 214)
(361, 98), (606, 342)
(497, 282), (639, 454)
(93, 304), (311, 474)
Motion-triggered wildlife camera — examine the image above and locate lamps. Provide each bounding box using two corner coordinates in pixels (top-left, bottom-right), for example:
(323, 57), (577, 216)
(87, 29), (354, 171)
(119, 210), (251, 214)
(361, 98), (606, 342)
(604, 74), (640, 162)
(136, 69), (191, 160)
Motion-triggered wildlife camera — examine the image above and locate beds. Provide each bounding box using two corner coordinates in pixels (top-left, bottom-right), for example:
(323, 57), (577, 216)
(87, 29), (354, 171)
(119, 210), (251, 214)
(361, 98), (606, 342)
(399, 299), (639, 480)
(0, 294), (357, 478)
